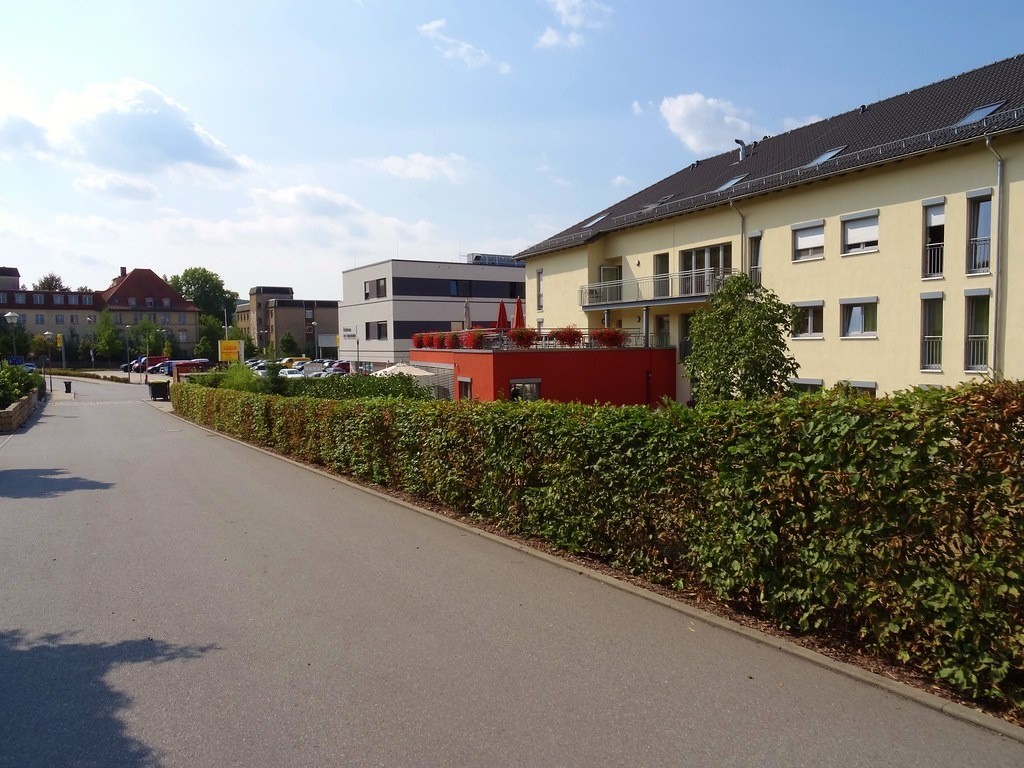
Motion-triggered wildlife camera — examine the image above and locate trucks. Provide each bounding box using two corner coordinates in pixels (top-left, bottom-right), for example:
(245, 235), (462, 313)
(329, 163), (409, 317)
(132, 356), (168, 373)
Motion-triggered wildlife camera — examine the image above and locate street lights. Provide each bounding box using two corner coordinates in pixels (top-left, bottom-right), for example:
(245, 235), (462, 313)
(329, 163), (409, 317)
(260, 330), (268, 359)
(87, 318), (94, 368)
(4, 312), (19, 370)
(126, 325), (131, 382)
(222, 325), (233, 371)
(44, 331), (54, 393)
(157, 328), (166, 357)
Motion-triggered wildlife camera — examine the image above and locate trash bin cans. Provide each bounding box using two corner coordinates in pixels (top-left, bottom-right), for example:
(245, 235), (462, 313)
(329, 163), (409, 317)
(147, 380), (170, 401)
(63, 381), (72, 393)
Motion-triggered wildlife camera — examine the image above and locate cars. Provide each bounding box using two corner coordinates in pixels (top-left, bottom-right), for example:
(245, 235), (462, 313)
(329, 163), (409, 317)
(120, 360), (139, 372)
(245, 357), (364, 378)
(146, 358), (209, 376)
